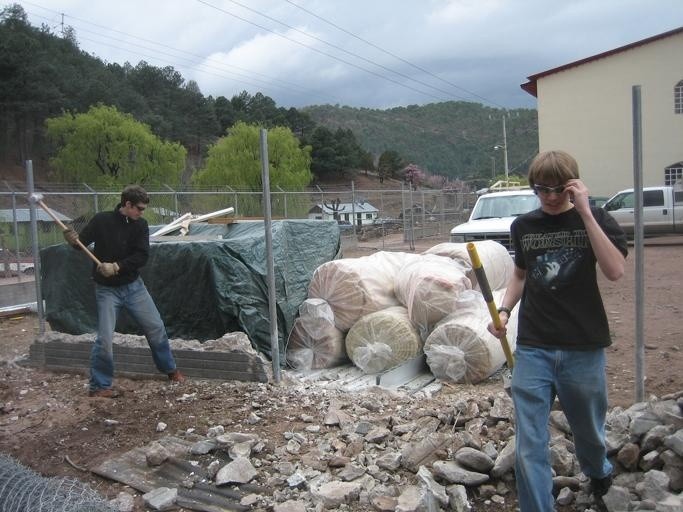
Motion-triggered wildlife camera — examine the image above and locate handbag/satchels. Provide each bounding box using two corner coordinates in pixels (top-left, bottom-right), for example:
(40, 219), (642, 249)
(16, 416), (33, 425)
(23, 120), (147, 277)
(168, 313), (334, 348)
(497, 306), (511, 318)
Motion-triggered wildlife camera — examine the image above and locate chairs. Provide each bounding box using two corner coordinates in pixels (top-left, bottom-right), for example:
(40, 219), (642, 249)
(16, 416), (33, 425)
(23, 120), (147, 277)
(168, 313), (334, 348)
(88, 388), (119, 397)
(590, 475), (613, 511)
(167, 368), (189, 386)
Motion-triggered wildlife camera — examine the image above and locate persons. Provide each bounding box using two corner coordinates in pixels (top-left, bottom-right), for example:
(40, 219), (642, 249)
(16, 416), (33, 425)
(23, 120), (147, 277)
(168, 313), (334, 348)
(61, 183), (185, 398)
(483, 149), (630, 512)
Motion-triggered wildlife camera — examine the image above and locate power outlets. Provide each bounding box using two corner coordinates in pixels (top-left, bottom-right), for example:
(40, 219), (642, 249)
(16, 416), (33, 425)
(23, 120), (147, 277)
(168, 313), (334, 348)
(602, 187), (683, 240)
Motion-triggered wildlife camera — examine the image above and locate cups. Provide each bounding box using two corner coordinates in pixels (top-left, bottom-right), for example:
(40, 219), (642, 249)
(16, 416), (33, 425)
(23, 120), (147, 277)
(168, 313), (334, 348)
(132, 203), (145, 211)
(531, 182), (566, 195)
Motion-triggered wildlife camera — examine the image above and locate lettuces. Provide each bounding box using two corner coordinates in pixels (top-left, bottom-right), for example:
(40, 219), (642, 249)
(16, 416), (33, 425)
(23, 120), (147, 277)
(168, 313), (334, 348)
(29, 193), (102, 265)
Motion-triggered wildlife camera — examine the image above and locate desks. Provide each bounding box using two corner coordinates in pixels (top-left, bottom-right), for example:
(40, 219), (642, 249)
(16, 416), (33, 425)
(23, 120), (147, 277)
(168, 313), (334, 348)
(493, 144), (509, 187)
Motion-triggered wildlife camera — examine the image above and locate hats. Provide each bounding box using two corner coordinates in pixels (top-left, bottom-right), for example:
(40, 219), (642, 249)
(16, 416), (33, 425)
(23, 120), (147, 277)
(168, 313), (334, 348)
(448, 190), (542, 259)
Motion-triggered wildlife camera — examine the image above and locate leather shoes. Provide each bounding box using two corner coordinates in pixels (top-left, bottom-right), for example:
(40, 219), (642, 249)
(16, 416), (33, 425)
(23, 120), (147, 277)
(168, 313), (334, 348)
(96, 262), (119, 278)
(62, 226), (79, 246)
